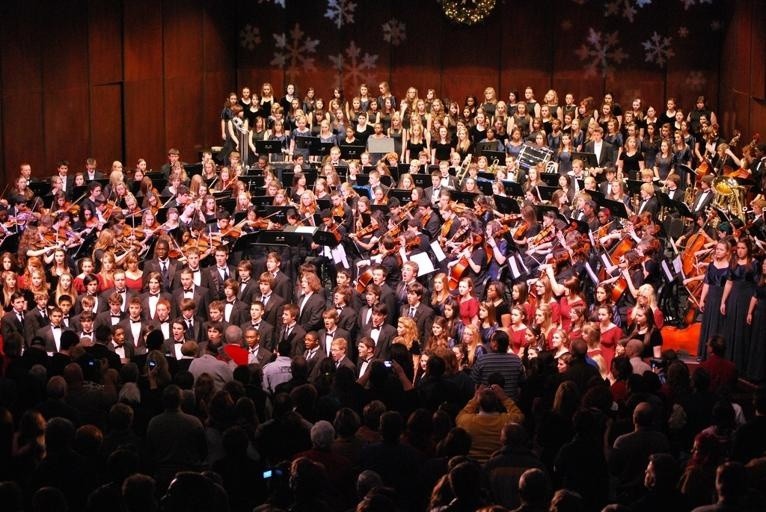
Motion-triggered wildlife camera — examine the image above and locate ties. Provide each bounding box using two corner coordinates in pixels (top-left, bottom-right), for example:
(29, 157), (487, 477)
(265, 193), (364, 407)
(222, 268), (228, 281)
(308, 351), (312, 360)
(285, 328), (289, 340)
(163, 262), (167, 276)
(188, 319), (195, 337)
(42, 310), (47, 320)
(19, 313), (25, 326)
(409, 308), (415, 319)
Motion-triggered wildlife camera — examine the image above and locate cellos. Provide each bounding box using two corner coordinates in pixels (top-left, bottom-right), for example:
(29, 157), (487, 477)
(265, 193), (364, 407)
(694, 133), (715, 183)
(447, 245), (474, 293)
(352, 268), (373, 295)
(598, 221), (642, 283)
(607, 256), (645, 305)
(688, 242), (721, 280)
(681, 214), (715, 275)
(730, 133), (760, 178)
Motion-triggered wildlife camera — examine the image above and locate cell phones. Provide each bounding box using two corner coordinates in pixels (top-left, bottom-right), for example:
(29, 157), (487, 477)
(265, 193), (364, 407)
(149, 361), (158, 366)
(88, 361), (95, 366)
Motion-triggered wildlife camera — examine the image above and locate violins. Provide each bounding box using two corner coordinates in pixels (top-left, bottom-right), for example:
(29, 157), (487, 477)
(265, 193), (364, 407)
(531, 224), (555, 245)
(331, 204), (344, 216)
(451, 220), (470, 244)
(64, 203), (80, 213)
(382, 227), (404, 238)
(329, 220), (345, 242)
(352, 224), (379, 239)
(447, 234), (471, 259)
(439, 217), (452, 242)
(512, 220), (530, 242)
(220, 224), (240, 238)
(249, 219), (280, 230)
(121, 224), (152, 237)
(181, 239), (216, 252)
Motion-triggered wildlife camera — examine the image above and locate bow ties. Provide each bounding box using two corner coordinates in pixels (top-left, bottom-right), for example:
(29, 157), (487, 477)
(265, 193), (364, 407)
(114, 345), (123, 349)
(184, 289), (193, 293)
(133, 319), (141, 323)
(111, 315), (121, 318)
(63, 316), (69, 319)
(251, 324), (259, 327)
(193, 269), (200, 273)
(261, 296), (266, 303)
(326, 332), (334, 337)
(361, 358), (368, 364)
(55, 325), (60, 328)
(335, 306), (342, 310)
(174, 340), (184, 344)
(372, 325), (380, 330)
(333, 359), (339, 364)
(118, 290), (125, 294)
(226, 300), (233, 304)
(241, 280), (247, 284)
(368, 304), (374, 309)
(83, 332), (92, 336)
(149, 293), (158, 298)
(160, 319), (170, 325)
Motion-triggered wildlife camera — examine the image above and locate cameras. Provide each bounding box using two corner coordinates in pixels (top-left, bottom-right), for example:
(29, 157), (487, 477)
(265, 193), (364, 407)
(372, 360), (392, 370)
(261, 460), (290, 480)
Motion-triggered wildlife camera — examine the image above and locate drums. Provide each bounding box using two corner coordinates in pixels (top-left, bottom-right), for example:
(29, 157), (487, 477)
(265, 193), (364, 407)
(516, 144), (552, 170)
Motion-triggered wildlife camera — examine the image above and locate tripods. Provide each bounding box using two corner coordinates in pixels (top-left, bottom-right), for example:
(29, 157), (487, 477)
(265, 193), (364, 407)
(309, 143), (335, 157)
(265, 206), (299, 227)
(256, 140), (281, 153)
(238, 175), (265, 192)
(282, 168), (317, 184)
(341, 145), (365, 160)
(370, 205), (388, 214)
(295, 135), (321, 150)
(311, 230), (339, 258)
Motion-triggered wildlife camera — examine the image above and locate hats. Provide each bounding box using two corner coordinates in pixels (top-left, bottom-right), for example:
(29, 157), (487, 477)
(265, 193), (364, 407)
(30, 336), (46, 346)
